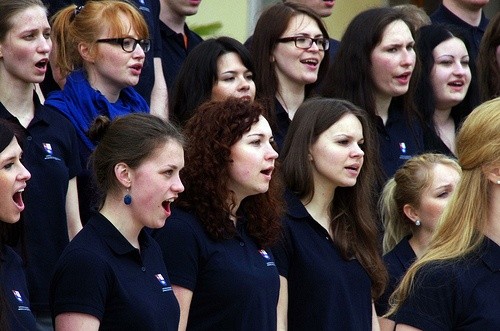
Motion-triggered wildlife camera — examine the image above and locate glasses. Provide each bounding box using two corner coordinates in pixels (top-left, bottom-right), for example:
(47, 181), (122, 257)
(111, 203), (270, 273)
(96, 36), (151, 53)
(275, 35), (330, 51)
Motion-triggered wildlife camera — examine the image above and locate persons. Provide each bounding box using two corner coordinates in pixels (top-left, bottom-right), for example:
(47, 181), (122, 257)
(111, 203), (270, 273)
(374, 154), (464, 331)
(0, 0), (53, 157)
(314, 8), (422, 196)
(258, 97), (381, 331)
(157, 96), (280, 331)
(52, 114), (185, 331)
(170, 37), (262, 129)
(475, 12), (500, 104)
(131, 0), (204, 124)
(242, 0), (341, 57)
(38, 1), (152, 190)
(0, 119), (41, 331)
(396, 97), (500, 331)
(380, 23), (476, 184)
(251, 2), (330, 164)
(425, 1), (492, 52)
